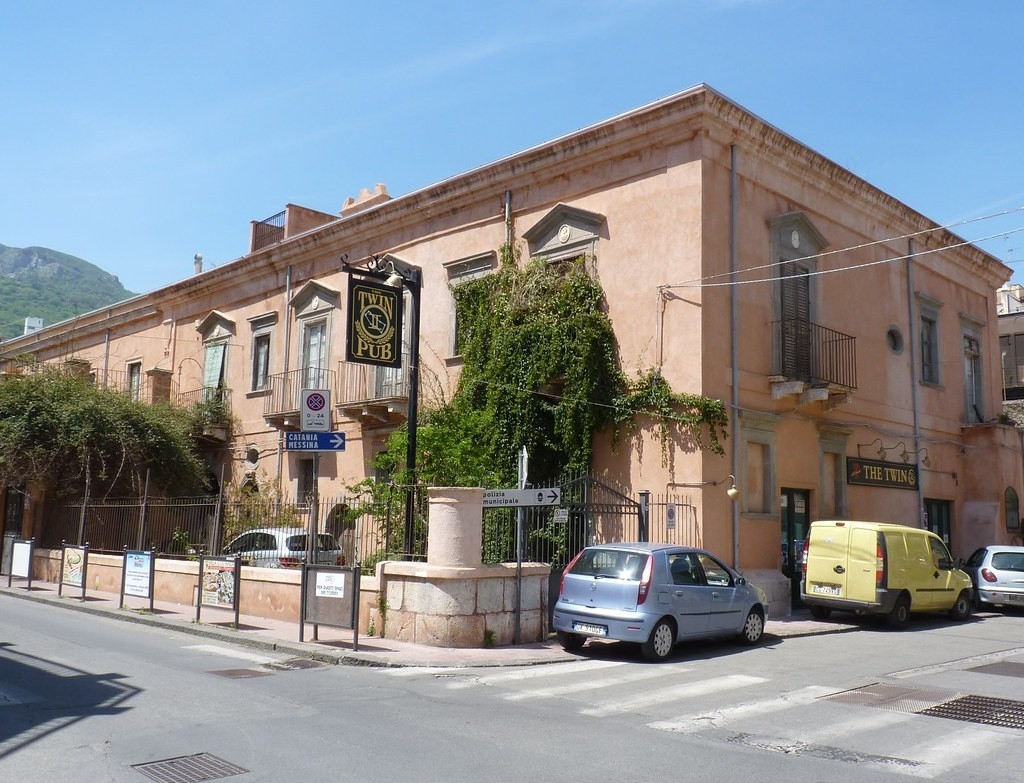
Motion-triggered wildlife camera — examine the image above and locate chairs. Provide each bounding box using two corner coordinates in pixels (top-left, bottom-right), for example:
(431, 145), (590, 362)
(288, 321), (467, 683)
(671, 559), (693, 585)
(626, 556), (640, 577)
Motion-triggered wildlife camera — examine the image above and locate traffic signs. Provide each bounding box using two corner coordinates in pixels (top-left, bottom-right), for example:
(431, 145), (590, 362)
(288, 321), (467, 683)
(286, 432), (346, 452)
(483, 488), (561, 506)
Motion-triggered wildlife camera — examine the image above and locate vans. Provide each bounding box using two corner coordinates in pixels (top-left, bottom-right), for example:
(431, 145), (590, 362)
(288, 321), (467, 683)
(799, 520), (973, 631)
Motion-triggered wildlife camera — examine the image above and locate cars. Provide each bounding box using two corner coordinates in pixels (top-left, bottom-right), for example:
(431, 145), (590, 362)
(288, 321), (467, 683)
(222, 527), (345, 568)
(552, 543), (768, 663)
(959, 545), (1024, 612)
